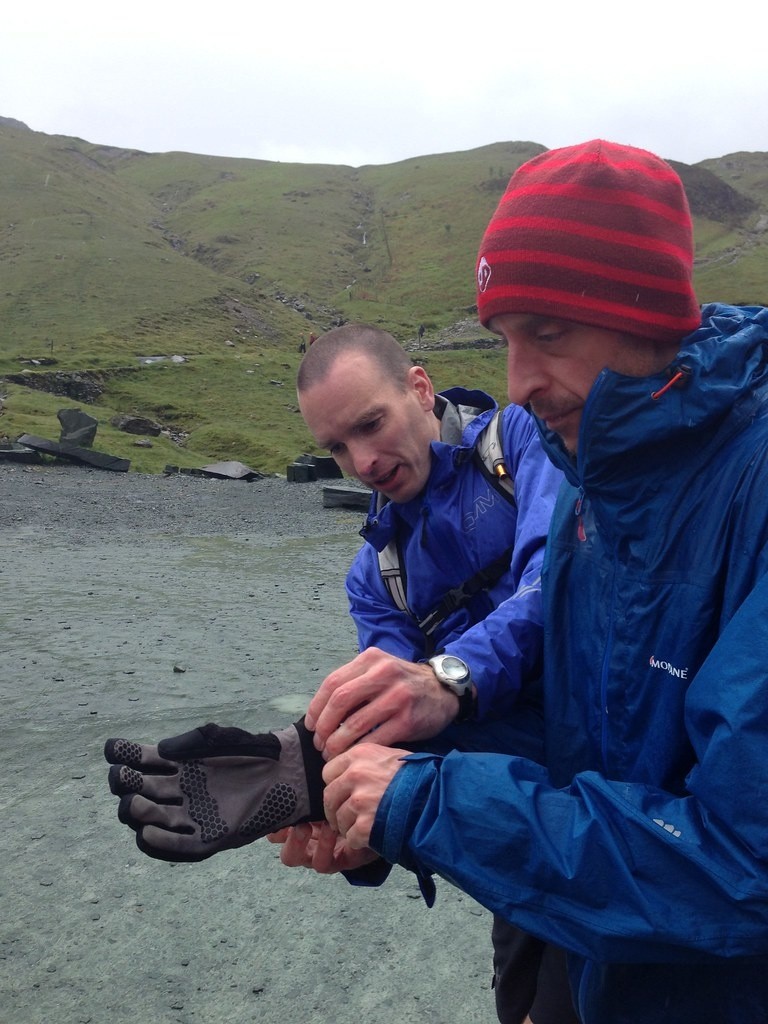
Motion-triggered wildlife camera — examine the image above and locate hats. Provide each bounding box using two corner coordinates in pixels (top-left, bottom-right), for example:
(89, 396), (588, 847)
(476, 138), (701, 340)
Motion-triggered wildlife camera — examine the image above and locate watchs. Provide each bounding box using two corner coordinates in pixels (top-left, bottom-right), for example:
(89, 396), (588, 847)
(423, 653), (480, 726)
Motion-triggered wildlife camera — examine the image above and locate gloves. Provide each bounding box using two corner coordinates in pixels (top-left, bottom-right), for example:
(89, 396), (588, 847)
(105, 714), (327, 862)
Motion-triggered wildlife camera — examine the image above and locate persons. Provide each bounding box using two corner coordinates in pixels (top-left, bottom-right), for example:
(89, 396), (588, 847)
(266, 323), (568, 1023)
(103, 138), (767, 1024)
